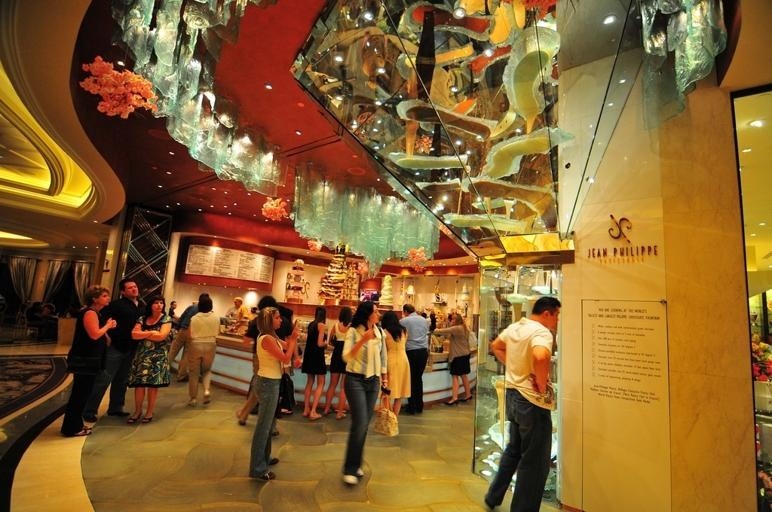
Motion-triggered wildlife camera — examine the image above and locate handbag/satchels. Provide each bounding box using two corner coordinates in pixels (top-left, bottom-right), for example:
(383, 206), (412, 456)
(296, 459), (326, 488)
(372, 409), (399, 437)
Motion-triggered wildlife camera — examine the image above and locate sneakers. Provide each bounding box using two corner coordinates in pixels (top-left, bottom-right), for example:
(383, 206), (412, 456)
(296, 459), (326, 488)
(237, 406), (363, 485)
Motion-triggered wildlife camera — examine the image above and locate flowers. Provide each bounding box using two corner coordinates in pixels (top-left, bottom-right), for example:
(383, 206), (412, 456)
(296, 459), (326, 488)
(261, 197), (288, 222)
(750, 334), (772, 381)
(408, 246), (427, 272)
(307, 240), (322, 254)
(78, 56), (158, 120)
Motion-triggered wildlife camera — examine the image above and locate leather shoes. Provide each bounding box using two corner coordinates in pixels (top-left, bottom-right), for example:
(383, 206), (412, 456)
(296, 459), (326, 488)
(69, 391), (210, 437)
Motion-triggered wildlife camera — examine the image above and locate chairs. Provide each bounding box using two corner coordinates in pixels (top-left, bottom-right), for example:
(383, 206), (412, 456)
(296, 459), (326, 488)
(24, 307), (38, 337)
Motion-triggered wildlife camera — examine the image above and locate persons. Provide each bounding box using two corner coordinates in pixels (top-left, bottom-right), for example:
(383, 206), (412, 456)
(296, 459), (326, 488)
(166, 293), (219, 405)
(249, 307), (299, 480)
(342, 301), (389, 486)
(61, 279), (173, 437)
(484, 296), (561, 512)
(432, 312), (472, 406)
(26, 302), (56, 338)
(222, 294), (434, 420)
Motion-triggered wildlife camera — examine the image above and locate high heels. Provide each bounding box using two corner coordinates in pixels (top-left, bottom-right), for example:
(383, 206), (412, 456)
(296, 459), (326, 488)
(444, 399), (458, 407)
(459, 396), (472, 402)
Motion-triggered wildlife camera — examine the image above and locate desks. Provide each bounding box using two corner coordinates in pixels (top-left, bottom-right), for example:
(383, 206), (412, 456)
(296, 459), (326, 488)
(41, 315), (78, 345)
